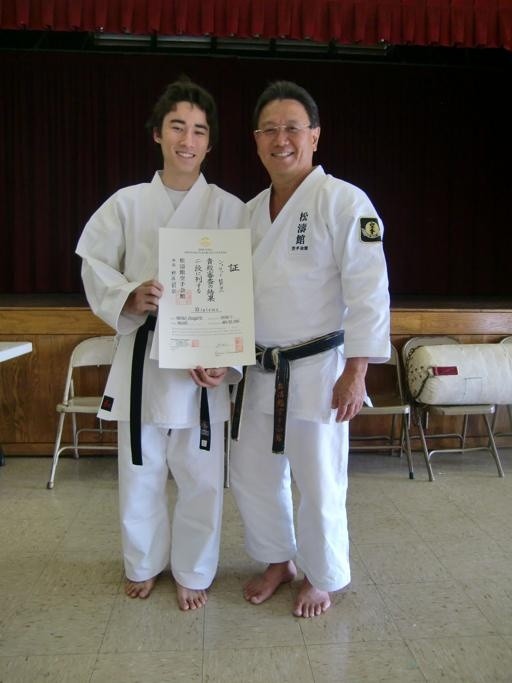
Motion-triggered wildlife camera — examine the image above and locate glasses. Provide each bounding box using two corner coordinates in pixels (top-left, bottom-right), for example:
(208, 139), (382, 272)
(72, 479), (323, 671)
(252, 125), (312, 138)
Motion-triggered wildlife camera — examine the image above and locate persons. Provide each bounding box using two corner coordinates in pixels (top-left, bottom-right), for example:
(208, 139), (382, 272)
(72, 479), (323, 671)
(75, 82), (252, 613)
(227, 79), (392, 618)
